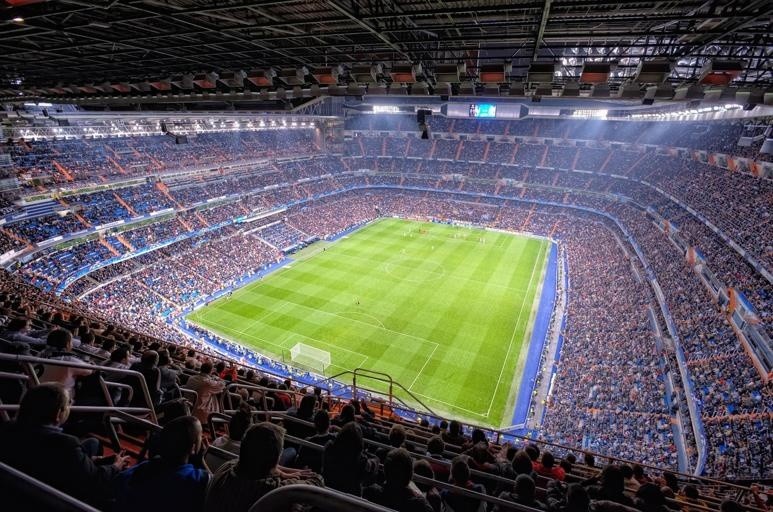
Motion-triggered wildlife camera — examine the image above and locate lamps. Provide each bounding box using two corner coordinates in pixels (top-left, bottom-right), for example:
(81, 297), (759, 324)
(12, 5), (27, 23)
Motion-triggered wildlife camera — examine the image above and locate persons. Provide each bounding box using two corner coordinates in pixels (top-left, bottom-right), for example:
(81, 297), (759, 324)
(2, 101), (773, 511)
